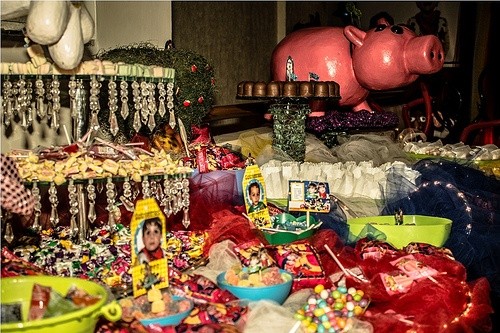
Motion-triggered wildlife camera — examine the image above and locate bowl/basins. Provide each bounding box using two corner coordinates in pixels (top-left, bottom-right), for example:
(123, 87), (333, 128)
(347, 215), (453, 250)
(139, 295), (194, 328)
(1, 275), (123, 333)
(259, 213), (321, 246)
(217, 269), (293, 306)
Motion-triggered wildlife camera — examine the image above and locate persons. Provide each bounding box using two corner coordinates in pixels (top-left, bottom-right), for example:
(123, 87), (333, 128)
(137, 219), (164, 263)
(308, 183), (329, 199)
(248, 182), (265, 212)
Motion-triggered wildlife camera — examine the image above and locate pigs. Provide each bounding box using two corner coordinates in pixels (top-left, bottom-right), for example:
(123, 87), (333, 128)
(265, 17), (445, 119)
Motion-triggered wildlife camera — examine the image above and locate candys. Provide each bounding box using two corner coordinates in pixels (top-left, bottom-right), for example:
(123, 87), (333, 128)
(8, 124), (248, 185)
(0, 221), (448, 333)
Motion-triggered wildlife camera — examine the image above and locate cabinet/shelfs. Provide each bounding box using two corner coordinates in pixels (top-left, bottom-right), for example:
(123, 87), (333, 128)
(1, 73), (194, 243)
